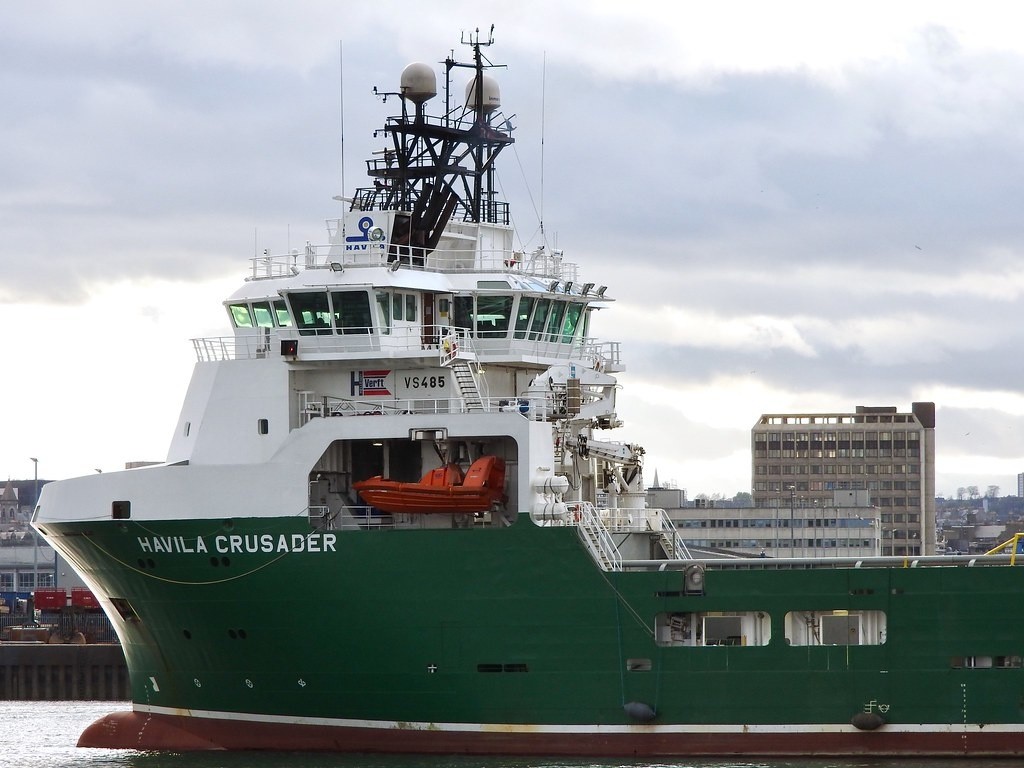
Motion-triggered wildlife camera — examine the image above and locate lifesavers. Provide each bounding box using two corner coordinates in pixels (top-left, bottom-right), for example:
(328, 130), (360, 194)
(447, 341), (458, 360)
(574, 505), (582, 522)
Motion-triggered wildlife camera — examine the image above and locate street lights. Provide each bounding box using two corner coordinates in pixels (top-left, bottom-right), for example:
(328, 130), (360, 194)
(30, 457), (38, 590)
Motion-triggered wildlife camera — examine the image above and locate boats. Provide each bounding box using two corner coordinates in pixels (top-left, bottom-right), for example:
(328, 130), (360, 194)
(29, 18), (1023, 754)
